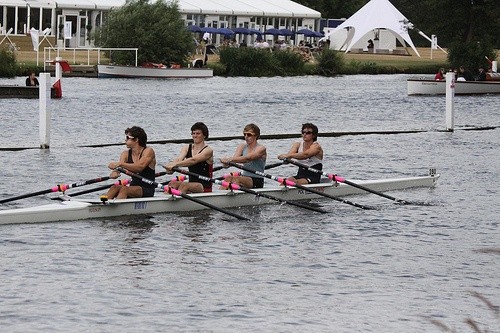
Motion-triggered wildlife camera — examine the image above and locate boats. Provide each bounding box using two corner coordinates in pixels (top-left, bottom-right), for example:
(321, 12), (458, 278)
(407, 77), (500, 95)
(0, 169), (441, 225)
(0, 84), (61, 99)
(97, 63), (214, 78)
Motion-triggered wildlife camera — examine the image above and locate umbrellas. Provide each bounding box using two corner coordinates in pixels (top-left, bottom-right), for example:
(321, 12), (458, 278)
(183, 25), (325, 44)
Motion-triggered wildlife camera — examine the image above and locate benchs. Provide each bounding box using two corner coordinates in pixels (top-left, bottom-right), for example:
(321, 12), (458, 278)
(376, 49), (389, 54)
(368, 48), (374, 53)
(351, 49), (363, 53)
(393, 50), (405, 54)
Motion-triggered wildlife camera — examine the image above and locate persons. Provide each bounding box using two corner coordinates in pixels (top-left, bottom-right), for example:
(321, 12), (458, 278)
(436, 66), (486, 82)
(26, 73), (40, 88)
(105, 126), (156, 199)
(218, 124), (267, 190)
(202, 36), (321, 53)
(278, 123), (323, 186)
(161, 121), (213, 195)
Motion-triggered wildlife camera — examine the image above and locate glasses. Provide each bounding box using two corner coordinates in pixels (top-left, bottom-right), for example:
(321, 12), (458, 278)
(125, 135), (134, 140)
(244, 132), (256, 137)
(302, 130), (312, 135)
